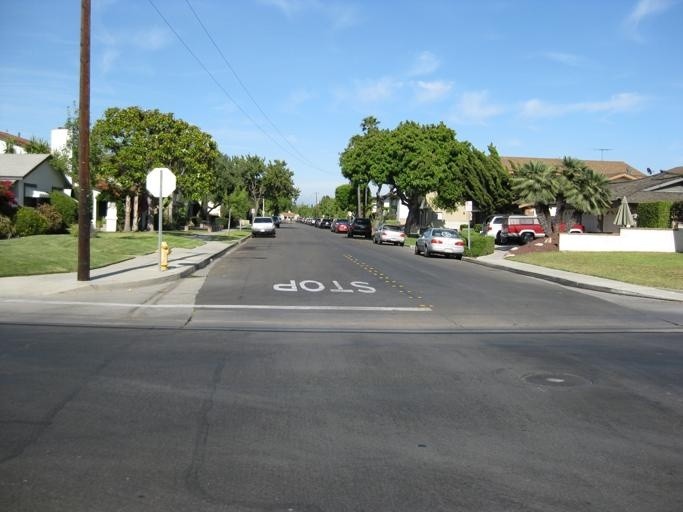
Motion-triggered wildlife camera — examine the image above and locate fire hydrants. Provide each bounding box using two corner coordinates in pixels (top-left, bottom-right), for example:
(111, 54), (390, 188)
(161, 241), (170, 270)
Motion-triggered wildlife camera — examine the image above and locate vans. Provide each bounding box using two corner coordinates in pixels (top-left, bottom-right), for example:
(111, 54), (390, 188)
(482, 215), (586, 246)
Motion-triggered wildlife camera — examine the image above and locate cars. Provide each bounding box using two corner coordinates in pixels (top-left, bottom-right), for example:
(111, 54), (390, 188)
(297, 216), (371, 239)
(373, 224), (406, 246)
(415, 228), (466, 260)
(251, 216), (281, 237)
